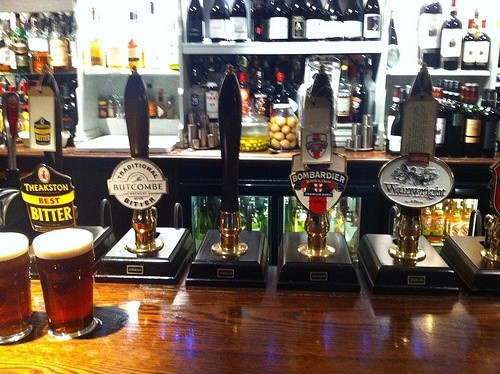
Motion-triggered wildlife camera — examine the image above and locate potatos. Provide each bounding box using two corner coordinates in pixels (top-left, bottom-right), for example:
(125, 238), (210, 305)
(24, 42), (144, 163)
(269, 116), (297, 148)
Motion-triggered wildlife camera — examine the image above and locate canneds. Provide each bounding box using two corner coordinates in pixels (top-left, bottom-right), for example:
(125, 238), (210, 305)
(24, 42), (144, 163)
(99, 101), (108, 118)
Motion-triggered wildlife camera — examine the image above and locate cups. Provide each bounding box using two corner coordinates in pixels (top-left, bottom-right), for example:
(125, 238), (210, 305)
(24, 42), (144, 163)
(400, 216), (422, 256)
(485, 220), (499, 258)
(133, 207), (156, 250)
(308, 211), (326, 254)
(31, 226), (105, 342)
(220, 211), (240, 255)
(0, 232), (36, 343)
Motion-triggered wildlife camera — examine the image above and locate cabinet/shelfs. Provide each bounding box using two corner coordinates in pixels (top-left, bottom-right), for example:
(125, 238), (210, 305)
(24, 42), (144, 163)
(0, 0), (500, 265)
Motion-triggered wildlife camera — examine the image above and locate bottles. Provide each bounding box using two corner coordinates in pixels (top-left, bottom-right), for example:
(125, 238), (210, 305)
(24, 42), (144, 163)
(0, 10), (78, 150)
(385, 0), (500, 160)
(419, 196), (478, 243)
(186, 52), (375, 154)
(95, 82), (177, 121)
(180, 0), (381, 43)
(87, 2), (178, 71)
